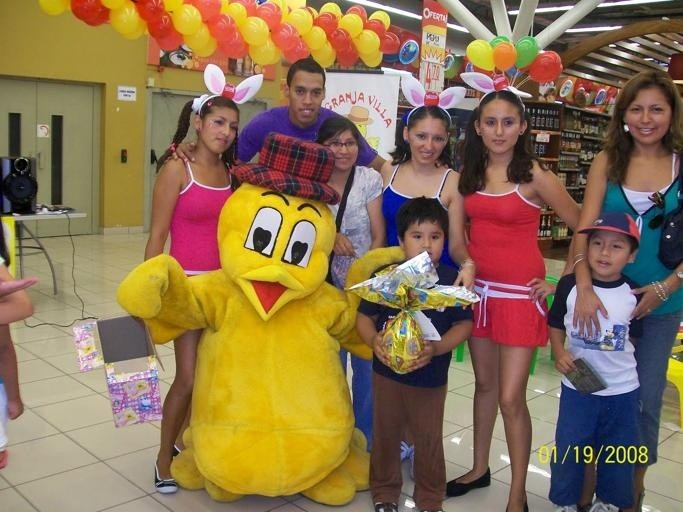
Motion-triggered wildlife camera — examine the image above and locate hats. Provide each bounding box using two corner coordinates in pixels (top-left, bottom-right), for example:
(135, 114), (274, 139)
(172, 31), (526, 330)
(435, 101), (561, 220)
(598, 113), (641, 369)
(576, 211), (640, 245)
(344, 106), (374, 125)
(231, 132), (340, 206)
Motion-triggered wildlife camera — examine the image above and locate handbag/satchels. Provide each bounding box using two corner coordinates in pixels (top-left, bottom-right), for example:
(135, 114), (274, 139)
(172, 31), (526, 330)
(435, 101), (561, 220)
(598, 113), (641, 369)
(647, 150), (683, 269)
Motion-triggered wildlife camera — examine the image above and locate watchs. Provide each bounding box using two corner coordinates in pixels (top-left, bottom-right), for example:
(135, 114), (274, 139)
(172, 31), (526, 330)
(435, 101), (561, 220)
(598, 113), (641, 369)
(673, 270), (683, 279)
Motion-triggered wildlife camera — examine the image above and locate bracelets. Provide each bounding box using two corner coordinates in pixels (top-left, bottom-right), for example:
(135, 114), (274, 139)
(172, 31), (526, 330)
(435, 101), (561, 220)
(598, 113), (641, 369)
(570, 252), (586, 268)
(651, 279), (670, 302)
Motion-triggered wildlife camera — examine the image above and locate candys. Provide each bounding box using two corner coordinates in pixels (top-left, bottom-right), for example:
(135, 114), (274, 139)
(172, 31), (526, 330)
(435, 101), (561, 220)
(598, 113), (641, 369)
(348, 251), (482, 375)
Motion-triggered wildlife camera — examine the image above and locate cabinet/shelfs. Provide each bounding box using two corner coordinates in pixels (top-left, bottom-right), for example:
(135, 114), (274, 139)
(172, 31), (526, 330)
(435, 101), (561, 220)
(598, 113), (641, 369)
(553, 105), (612, 246)
(521, 99), (565, 259)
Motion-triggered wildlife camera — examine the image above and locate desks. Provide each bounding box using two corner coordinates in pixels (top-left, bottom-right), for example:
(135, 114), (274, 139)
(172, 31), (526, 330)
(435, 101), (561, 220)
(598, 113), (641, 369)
(0, 203), (87, 295)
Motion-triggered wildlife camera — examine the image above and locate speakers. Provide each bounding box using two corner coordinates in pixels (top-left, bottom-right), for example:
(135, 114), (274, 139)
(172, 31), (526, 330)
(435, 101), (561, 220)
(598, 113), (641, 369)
(0, 157), (38, 215)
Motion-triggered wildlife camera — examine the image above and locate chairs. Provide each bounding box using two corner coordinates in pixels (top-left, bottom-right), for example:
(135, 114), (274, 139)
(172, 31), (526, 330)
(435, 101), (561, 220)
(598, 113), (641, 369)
(664, 332), (683, 426)
(529, 274), (560, 374)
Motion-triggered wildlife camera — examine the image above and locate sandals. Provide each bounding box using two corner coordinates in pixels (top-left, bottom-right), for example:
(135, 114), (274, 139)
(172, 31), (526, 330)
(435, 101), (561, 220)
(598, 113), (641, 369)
(152, 461), (177, 494)
(421, 509), (442, 512)
(446, 467), (491, 497)
(504, 502), (528, 512)
(172, 444), (180, 458)
(374, 497), (398, 512)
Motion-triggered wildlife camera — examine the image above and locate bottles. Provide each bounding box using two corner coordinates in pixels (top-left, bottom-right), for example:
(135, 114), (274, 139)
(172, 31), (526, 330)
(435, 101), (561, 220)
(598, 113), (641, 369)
(554, 110), (557, 129)
(581, 142), (600, 161)
(573, 111), (582, 130)
(533, 136), (546, 156)
(561, 156), (578, 168)
(541, 109), (545, 129)
(547, 215), (551, 237)
(557, 110), (560, 129)
(556, 223), (569, 238)
(531, 108), (536, 128)
(585, 118), (609, 137)
(546, 109), (549, 129)
(540, 215), (546, 237)
(536, 108), (541, 128)
(527, 108), (530, 114)
(561, 132), (581, 152)
(550, 110), (554, 129)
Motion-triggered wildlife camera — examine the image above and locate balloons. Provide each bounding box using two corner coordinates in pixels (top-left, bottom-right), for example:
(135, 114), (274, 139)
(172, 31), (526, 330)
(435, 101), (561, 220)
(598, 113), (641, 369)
(134, 0), (400, 67)
(490, 34), (511, 48)
(529, 51), (561, 78)
(492, 42), (517, 72)
(506, 66), (523, 78)
(466, 39), (494, 71)
(465, 62), (473, 72)
(70, 1), (110, 27)
(472, 64), (492, 76)
(535, 64), (563, 82)
(41, 1), (65, 15)
(102, 1), (147, 39)
(514, 36), (538, 69)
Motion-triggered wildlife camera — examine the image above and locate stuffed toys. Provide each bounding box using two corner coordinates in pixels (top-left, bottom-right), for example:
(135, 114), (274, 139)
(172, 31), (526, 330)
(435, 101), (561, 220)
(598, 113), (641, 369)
(115, 132), (406, 506)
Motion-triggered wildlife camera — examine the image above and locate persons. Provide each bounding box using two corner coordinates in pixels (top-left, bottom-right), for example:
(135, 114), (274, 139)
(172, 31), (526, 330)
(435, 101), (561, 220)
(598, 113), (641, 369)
(445, 90), (581, 512)
(142, 96), (241, 495)
(161, 58), (448, 187)
(546, 211), (644, 512)
(573, 70), (683, 512)
(0, 260), (38, 468)
(356, 196), (474, 512)
(314, 116), (385, 454)
(380, 104), (475, 480)
